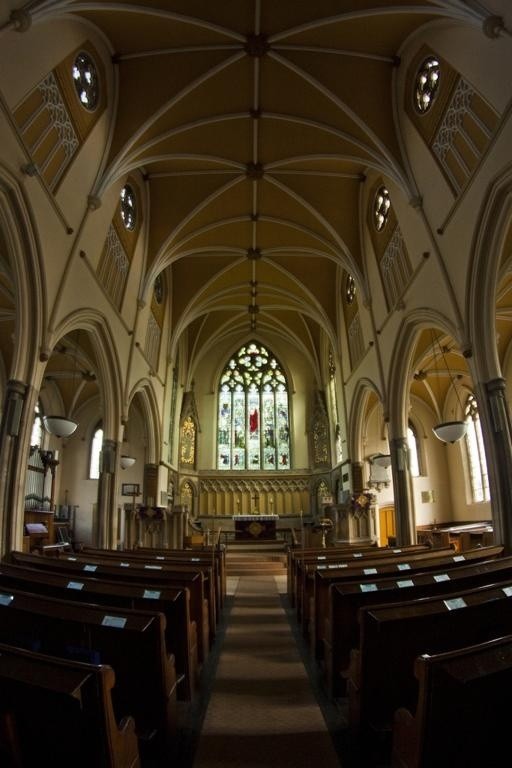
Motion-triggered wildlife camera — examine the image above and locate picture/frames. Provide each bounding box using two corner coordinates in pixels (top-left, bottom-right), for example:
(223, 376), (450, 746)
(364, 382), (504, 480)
(122, 483), (140, 496)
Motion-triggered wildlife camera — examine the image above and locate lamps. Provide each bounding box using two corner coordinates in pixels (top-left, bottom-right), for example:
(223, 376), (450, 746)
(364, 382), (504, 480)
(42, 329), (78, 441)
(431, 329), (469, 445)
(373, 394), (394, 469)
(119, 398), (136, 471)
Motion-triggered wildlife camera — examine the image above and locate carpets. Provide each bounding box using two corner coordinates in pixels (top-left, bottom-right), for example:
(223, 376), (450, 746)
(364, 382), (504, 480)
(184, 574), (351, 767)
